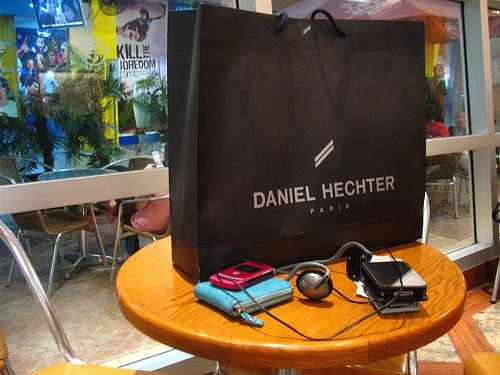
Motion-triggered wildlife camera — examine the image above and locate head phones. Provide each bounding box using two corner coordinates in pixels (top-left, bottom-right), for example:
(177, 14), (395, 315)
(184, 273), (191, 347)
(275, 242), (372, 301)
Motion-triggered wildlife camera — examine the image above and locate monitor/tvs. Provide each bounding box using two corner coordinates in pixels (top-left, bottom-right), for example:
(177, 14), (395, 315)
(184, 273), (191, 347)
(32, 0), (85, 29)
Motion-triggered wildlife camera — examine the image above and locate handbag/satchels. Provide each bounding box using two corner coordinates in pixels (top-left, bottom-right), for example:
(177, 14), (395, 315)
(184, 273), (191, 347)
(168, 4), (427, 282)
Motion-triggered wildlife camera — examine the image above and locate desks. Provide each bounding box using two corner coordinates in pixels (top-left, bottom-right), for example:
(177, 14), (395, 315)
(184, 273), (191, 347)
(38, 168), (123, 279)
(117, 234), (468, 375)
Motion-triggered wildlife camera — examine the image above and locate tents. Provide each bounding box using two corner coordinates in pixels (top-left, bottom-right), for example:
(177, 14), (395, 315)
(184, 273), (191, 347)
(273, 0), (500, 111)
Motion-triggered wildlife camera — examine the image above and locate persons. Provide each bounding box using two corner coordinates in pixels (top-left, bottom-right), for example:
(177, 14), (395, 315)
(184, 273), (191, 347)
(423, 103), (466, 180)
(39, 0), (83, 26)
(119, 131), (171, 264)
(0, 76), (18, 119)
(425, 63), (448, 124)
(15, 32), (68, 100)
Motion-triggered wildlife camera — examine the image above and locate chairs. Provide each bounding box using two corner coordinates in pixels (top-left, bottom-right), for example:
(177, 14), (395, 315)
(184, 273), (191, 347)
(0, 156), (153, 300)
(409, 151), (500, 375)
(0, 218), (171, 375)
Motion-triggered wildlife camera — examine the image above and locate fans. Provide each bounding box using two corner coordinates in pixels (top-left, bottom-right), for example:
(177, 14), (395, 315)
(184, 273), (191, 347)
(82, 0), (129, 16)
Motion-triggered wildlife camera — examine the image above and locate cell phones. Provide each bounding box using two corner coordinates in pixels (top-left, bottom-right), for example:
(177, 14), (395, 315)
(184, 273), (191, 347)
(360, 261), (428, 301)
(209, 261), (275, 291)
